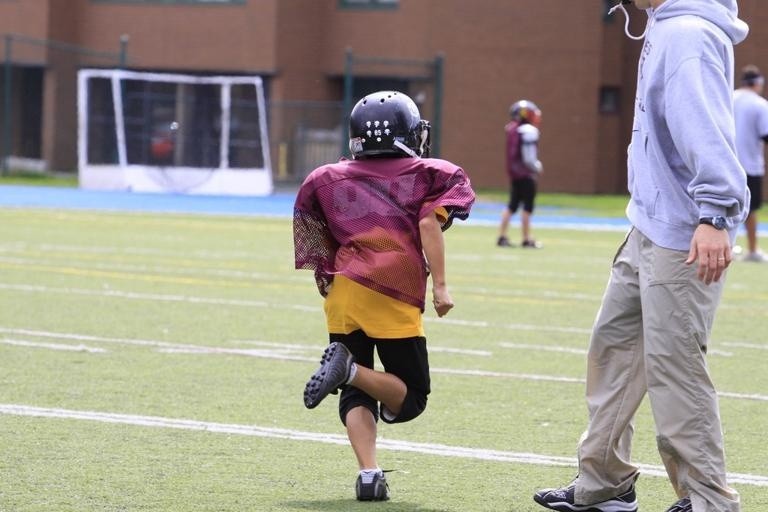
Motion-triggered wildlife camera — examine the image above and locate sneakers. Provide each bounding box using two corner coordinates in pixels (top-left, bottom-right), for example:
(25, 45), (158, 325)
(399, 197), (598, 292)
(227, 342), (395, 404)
(664, 490), (741, 512)
(356, 469), (391, 500)
(531, 472), (640, 512)
(499, 237), (542, 249)
(303, 342), (354, 409)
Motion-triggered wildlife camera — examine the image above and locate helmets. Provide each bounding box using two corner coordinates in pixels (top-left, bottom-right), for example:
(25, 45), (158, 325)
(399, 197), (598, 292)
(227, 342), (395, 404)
(350, 89), (431, 160)
(510, 99), (542, 127)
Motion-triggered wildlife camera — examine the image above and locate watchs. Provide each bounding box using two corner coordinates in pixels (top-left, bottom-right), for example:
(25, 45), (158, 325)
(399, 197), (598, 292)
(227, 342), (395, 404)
(698, 215), (729, 230)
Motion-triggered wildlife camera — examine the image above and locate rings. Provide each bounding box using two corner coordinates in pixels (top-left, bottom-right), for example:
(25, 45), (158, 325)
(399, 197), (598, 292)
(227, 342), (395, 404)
(717, 257), (727, 261)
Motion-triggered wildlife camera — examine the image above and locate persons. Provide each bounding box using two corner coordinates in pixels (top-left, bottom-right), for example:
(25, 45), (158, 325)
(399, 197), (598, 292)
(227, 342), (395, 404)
(529, 0), (753, 512)
(730, 61), (768, 265)
(494, 95), (547, 251)
(290, 88), (477, 504)
